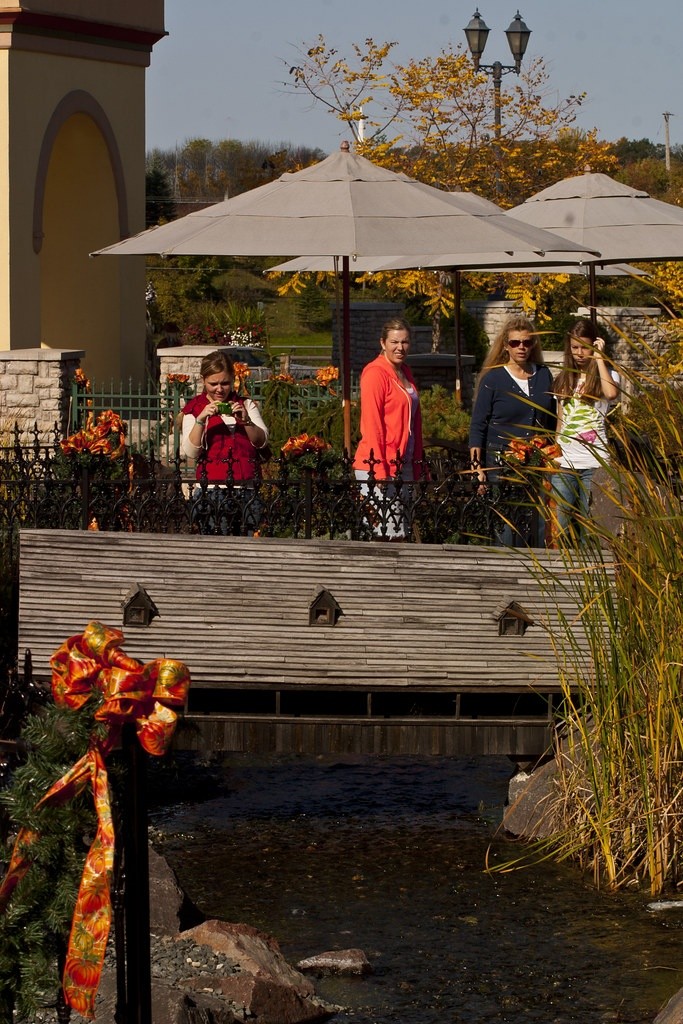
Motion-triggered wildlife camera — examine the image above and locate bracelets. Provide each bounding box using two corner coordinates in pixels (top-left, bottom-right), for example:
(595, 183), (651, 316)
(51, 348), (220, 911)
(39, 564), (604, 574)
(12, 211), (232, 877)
(195, 418), (204, 426)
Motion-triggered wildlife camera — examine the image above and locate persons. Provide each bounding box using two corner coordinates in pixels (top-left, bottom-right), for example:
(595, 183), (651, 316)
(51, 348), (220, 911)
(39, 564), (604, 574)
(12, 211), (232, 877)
(469, 317), (557, 547)
(353, 318), (430, 538)
(183, 351), (268, 537)
(554, 320), (620, 549)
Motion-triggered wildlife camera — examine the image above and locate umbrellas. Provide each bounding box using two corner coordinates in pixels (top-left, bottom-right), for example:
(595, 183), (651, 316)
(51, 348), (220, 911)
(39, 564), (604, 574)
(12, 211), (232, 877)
(89, 140), (683, 463)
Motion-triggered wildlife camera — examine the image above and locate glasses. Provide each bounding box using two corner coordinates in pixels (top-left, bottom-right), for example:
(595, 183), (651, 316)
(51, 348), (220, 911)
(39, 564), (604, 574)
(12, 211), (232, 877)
(505, 339), (533, 348)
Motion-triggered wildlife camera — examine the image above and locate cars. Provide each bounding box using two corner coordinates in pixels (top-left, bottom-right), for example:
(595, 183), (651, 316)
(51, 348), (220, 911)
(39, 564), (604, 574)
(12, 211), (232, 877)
(236, 346), (318, 381)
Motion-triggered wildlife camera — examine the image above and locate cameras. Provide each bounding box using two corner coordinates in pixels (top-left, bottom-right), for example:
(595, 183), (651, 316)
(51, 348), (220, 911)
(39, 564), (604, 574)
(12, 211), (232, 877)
(215, 402), (233, 414)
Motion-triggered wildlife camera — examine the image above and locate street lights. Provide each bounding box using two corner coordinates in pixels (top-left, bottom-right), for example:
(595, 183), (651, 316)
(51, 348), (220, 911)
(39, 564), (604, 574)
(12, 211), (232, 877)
(461, 7), (532, 202)
